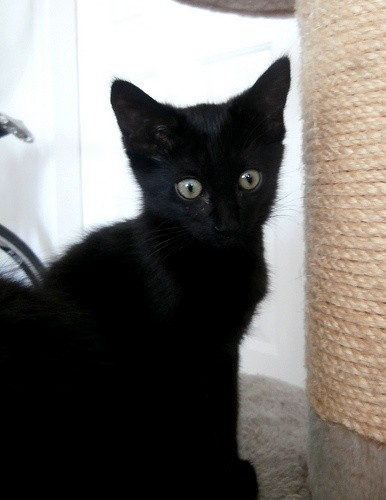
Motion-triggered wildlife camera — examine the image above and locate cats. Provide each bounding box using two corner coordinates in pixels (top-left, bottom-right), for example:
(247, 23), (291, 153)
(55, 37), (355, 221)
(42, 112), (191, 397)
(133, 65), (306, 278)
(0, 56), (292, 500)
